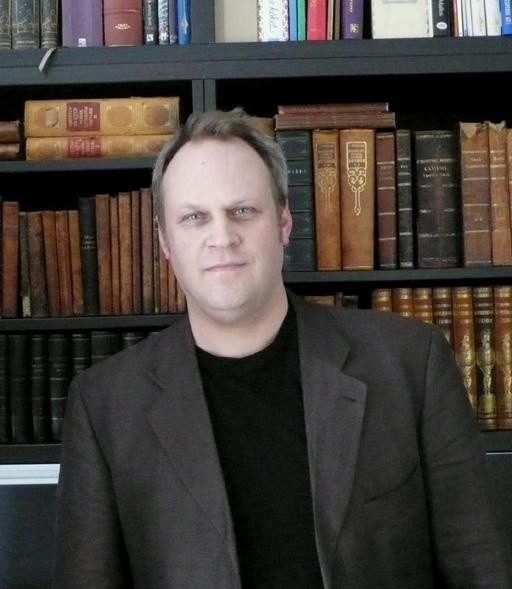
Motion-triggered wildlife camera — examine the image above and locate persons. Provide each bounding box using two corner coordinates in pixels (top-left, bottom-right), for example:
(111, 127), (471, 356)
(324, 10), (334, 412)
(47, 107), (512, 589)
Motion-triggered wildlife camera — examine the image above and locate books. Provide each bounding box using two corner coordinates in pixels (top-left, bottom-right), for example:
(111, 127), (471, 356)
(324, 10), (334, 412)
(248, 106), (511, 272)
(1, 97), (180, 161)
(1, 332), (147, 447)
(257, 1), (511, 41)
(0, 0), (215, 50)
(1, 188), (187, 317)
(298, 286), (511, 430)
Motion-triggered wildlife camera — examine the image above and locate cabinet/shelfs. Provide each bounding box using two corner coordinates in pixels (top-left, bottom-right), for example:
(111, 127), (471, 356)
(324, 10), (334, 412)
(202, 34), (512, 465)
(1, 44), (203, 466)
